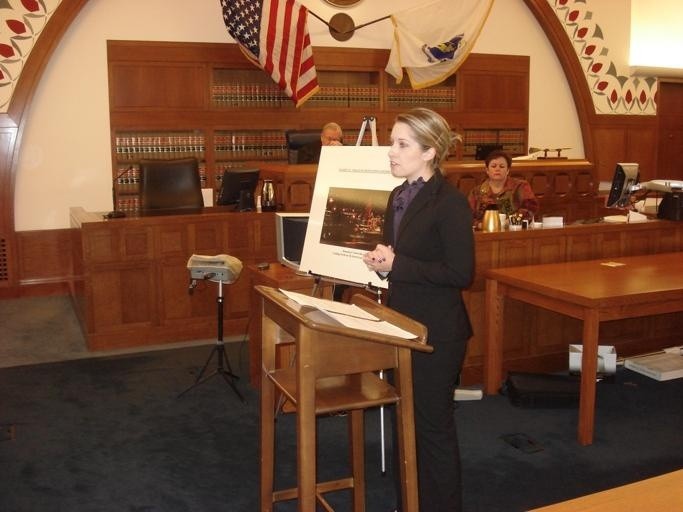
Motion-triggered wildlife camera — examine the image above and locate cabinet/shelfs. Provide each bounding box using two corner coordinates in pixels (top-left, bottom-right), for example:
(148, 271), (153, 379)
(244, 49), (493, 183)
(106, 39), (531, 216)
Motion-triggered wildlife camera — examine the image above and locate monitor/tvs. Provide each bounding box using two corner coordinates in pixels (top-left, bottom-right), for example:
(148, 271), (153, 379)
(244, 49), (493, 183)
(604, 163), (640, 209)
(215, 165), (260, 213)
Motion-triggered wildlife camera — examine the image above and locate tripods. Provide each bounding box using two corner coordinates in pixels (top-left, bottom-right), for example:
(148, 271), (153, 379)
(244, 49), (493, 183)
(178, 282), (251, 405)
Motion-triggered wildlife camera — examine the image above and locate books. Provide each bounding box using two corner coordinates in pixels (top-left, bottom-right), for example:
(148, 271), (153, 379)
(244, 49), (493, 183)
(450, 130), (524, 155)
(116, 132), (288, 161)
(199, 163), (244, 204)
(211, 82), (457, 109)
(117, 164), (140, 211)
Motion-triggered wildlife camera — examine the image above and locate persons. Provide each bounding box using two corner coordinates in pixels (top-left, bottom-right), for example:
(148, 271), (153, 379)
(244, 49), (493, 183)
(468, 151), (539, 231)
(364, 108), (477, 511)
(297, 123), (343, 165)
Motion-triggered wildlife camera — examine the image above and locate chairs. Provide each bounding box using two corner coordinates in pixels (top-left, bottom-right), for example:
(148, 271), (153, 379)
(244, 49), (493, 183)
(284, 128), (321, 163)
(138, 155), (205, 210)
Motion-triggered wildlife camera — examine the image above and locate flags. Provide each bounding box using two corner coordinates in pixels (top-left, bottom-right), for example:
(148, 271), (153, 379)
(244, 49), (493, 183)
(385, 0), (495, 91)
(220, 0), (320, 109)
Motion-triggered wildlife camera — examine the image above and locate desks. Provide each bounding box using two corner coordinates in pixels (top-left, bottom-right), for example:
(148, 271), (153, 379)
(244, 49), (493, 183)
(253, 284), (434, 512)
(248, 261), (333, 410)
(462, 221), (683, 365)
(486, 252), (683, 444)
(248, 158), (597, 219)
(66, 205), (312, 349)
